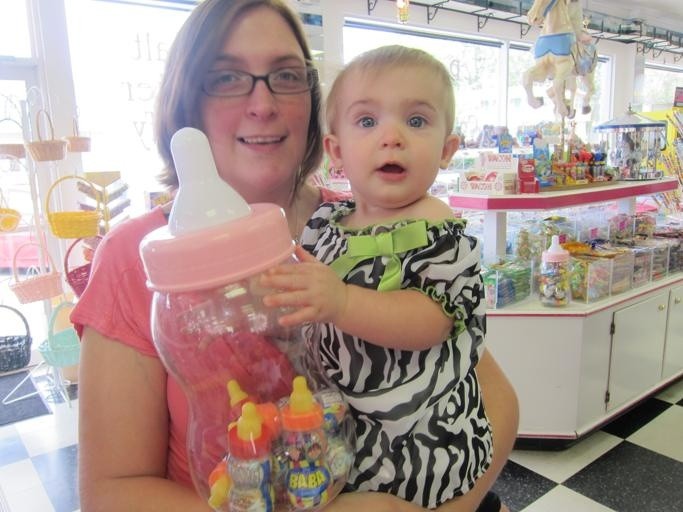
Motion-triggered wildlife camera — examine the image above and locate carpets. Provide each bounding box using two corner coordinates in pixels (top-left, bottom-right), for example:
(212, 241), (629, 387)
(0, 372), (48, 427)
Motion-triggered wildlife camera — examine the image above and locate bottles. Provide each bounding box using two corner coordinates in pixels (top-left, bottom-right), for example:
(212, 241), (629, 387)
(538, 234), (573, 307)
(139, 125), (360, 511)
(278, 375), (333, 510)
(219, 380), (261, 415)
(201, 452), (228, 508)
(225, 406), (276, 510)
(558, 160), (606, 180)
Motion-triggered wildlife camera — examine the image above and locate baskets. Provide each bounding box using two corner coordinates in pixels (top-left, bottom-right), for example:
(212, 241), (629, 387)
(46, 176), (101, 238)
(1, 305), (31, 373)
(63, 238), (104, 296)
(0, 110), (90, 162)
(2, 191), (23, 231)
(39, 304), (81, 365)
(12, 242), (61, 304)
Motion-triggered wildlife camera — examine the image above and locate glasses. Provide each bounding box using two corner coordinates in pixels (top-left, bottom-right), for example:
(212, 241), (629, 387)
(200, 66), (313, 95)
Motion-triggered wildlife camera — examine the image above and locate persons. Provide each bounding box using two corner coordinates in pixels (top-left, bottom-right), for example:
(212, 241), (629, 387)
(258, 43), (510, 512)
(70, 1), (520, 511)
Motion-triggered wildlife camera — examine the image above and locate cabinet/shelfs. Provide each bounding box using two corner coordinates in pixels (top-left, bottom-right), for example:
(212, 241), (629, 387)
(448, 176), (683, 437)
